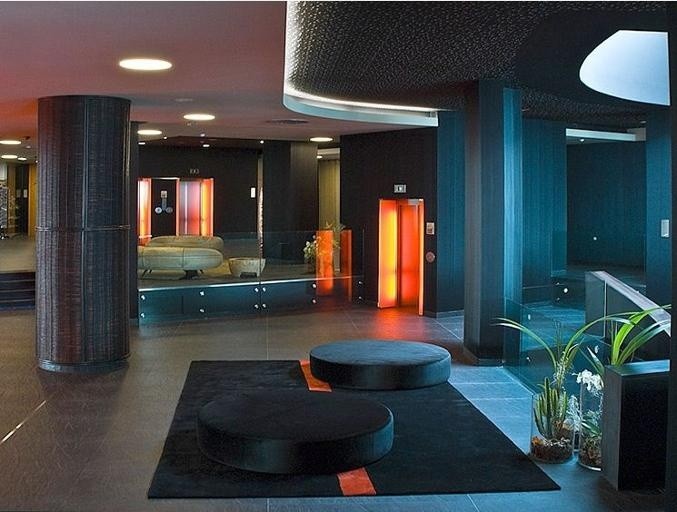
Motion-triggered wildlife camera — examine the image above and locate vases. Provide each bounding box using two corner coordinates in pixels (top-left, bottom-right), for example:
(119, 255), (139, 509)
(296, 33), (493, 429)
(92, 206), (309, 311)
(576, 385), (604, 472)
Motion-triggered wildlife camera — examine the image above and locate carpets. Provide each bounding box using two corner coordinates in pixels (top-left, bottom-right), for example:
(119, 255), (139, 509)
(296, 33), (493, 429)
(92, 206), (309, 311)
(147, 360), (562, 499)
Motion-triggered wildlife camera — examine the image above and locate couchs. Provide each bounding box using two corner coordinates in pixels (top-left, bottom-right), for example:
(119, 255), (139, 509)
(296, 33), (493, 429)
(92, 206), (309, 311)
(137, 230), (224, 284)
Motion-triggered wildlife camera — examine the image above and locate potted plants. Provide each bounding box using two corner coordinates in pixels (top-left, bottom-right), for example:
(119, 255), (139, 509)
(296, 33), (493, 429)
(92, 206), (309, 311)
(491, 302), (671, 465)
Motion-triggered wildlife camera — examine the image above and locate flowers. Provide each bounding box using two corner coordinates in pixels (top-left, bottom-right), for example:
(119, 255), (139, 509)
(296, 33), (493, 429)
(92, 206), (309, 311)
(572, 368), (604, 392)
